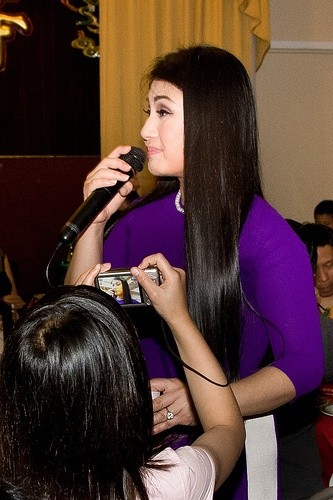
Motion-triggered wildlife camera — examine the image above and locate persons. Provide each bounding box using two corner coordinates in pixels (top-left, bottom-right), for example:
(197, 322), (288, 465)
(61, 43), (326, 500)
(282, 199), (333, 377)
(106, 275), (140, 305)
(0, 252), (246, 500)
(0, 252), (27, 342)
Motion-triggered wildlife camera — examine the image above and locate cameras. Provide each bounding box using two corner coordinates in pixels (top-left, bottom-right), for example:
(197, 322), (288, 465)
(94, 266), (161, 309)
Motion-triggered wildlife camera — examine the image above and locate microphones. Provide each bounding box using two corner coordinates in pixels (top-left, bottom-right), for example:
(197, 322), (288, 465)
(58, 146), (147, 246)
(111, 290), (115, 294)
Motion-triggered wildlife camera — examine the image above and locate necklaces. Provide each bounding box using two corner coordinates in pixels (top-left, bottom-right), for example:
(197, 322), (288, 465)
(175, 184), (185, 214)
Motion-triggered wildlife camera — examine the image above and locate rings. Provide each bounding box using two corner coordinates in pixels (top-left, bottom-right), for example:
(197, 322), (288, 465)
(166, 407), (174, 420)
